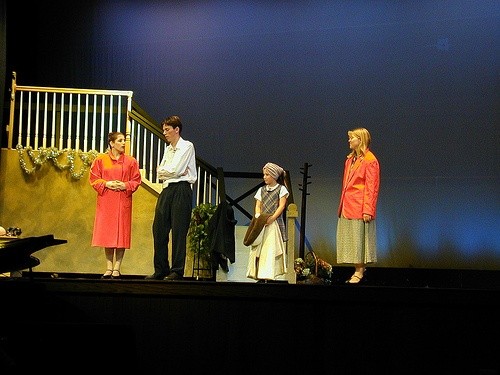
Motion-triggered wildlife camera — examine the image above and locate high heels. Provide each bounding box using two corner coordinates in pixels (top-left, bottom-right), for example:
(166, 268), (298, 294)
(349, 269), (366, 283)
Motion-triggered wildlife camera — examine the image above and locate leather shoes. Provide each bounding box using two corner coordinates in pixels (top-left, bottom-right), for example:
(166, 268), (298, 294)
(145, 270), (170, 280)
(163, 272), (183, 281)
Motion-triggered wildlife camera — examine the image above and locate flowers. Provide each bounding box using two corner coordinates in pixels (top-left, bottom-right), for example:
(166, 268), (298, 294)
(293, 251), (334, 286)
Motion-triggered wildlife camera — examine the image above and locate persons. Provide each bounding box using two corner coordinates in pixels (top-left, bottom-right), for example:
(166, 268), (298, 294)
(336, 127), (381, 285)
(254, 162), (290, 282)
(89, 132), (142, 279)
(145, 116), (197, 282)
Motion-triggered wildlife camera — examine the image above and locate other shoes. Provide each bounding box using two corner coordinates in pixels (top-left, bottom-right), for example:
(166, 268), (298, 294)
(112, 270), (121, 279)
(101, 270), (112, 279)
